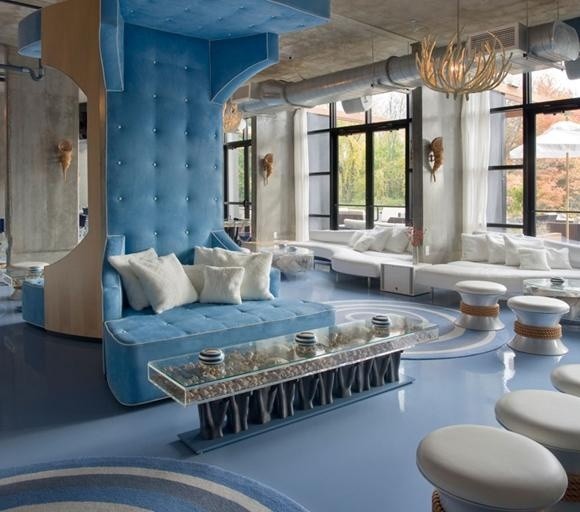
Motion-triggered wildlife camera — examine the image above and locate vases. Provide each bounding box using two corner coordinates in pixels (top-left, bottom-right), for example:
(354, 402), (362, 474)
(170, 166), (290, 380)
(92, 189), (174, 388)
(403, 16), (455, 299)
(412, 245), (419, 265)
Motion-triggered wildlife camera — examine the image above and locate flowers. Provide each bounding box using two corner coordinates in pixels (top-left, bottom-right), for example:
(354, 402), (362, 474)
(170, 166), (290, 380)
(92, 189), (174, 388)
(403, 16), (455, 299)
(405, 222), (428, 246)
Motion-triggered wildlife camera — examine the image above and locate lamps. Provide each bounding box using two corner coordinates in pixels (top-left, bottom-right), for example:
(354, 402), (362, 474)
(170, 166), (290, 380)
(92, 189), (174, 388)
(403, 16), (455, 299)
(221, 98), (242, 133)
(414, 0), (513, 101)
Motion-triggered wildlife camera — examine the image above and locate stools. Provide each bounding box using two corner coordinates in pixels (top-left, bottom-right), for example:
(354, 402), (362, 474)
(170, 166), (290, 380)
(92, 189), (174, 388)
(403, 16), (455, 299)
(454, 280), (508, 331)
(495, 389), (580, 499)
(550, 362), (580, 397)
(506, 296), (571, 356)
(415, 422), (570, 512)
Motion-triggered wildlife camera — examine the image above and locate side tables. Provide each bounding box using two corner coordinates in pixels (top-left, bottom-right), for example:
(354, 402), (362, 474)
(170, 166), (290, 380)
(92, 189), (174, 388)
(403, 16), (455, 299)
(521, 278), (580, 332)
(378, 262), (431, 296)
(258, 244), (314, 280)
(9, 261), (50, 301)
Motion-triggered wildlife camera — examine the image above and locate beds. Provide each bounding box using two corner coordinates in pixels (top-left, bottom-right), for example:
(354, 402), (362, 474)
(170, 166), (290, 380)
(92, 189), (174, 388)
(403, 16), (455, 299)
(99, 0), (334, 408)
(18, 0), (103, 339)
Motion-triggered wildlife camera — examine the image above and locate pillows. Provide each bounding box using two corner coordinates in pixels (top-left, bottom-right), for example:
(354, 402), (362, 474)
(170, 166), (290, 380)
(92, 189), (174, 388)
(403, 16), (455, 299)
(209, 247), (276, 301)
(198, 265), (246, 306)
(179, 261), (195, 285)
(106, 247), (159, 310)
(349, 225), (412, 254)
(129, 251), (200, 313)
(192, 244), (250, 287)
(461, 231), (572, 270)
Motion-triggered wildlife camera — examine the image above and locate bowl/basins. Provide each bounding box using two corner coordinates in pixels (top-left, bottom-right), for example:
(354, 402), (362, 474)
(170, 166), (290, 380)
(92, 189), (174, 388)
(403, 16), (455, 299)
(295, 332), (318, 345)
(29, 266), (43, 274)
(199, 347), (224, 365)
(550, 276), (565, 285)
(371, 315), (391, 327)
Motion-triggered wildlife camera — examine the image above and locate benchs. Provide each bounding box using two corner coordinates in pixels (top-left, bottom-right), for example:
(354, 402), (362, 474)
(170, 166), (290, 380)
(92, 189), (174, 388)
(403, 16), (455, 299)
(415, 239), (580, 305)
(285, 228), (411, 290)
(534, 214), (580, 240)
(338, 207), (406, 230)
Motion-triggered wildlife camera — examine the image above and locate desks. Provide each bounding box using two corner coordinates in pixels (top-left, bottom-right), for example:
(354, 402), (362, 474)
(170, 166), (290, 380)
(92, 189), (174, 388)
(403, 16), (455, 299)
(222, 218), (251, 243)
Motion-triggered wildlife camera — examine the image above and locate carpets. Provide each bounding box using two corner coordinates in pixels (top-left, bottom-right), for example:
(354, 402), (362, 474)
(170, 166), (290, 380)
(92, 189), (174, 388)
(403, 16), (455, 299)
(0, 457), (310, 512)
